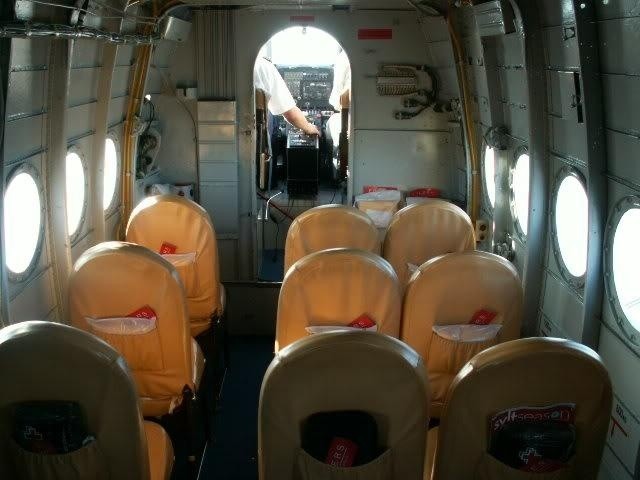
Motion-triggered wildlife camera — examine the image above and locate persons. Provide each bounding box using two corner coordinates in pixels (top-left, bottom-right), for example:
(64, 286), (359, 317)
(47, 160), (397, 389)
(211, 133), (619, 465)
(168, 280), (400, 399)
(323, 47), (351, 184)
(252, 54), (321, 190)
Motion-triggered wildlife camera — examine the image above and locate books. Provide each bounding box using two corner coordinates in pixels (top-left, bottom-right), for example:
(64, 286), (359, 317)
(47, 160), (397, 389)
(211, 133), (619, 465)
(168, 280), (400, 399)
(488, 402), (576, 454)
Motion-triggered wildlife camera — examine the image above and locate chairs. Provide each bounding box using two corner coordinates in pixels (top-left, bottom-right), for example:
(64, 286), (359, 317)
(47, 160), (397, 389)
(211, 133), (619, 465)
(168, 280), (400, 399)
(1, 321), (174, 480)
(425, 337), (612, 479)
(336, 89), (350, 183)
(255, 87), (273, 195)
(125, 195), (227, 402)
(67, 242), (204, 460)
(275, 199), (523, 418)
(259, 328), (428, 479)
